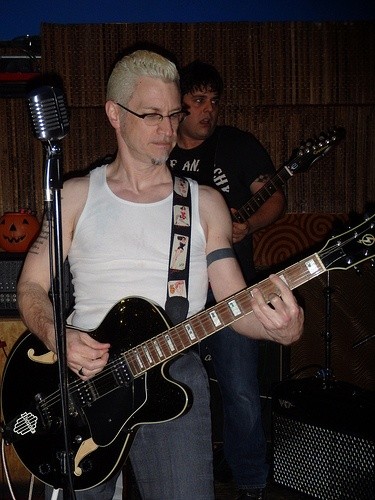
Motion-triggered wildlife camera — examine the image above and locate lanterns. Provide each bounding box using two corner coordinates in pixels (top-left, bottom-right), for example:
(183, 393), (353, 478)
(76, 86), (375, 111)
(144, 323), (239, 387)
(0, 209), (39, 252)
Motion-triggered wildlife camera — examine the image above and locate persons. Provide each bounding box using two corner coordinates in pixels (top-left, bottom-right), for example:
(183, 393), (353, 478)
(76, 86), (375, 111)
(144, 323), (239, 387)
(165, 59), (287, 500)
(16, 50), (305, 500)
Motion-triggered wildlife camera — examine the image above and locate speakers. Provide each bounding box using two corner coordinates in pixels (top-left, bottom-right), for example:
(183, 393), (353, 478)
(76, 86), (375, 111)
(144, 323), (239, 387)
(269, 376), (375, 500)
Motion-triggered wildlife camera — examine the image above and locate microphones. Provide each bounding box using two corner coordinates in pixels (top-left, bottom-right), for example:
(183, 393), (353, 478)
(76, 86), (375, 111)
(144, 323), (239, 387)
(24, 85), (70, 142)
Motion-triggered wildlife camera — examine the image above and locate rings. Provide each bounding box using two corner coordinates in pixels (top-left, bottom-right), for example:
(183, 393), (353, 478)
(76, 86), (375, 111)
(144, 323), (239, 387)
(78, 366), (85, 377)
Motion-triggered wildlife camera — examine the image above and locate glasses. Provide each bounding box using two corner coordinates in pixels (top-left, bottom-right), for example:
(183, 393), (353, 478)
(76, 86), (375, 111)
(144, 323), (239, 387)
(114, 102), (191, 126)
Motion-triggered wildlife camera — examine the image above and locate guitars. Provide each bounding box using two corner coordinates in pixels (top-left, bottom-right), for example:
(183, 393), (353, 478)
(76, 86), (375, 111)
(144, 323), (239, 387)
(1, 215), (375, 490)
(231, 125), (345, 223)
